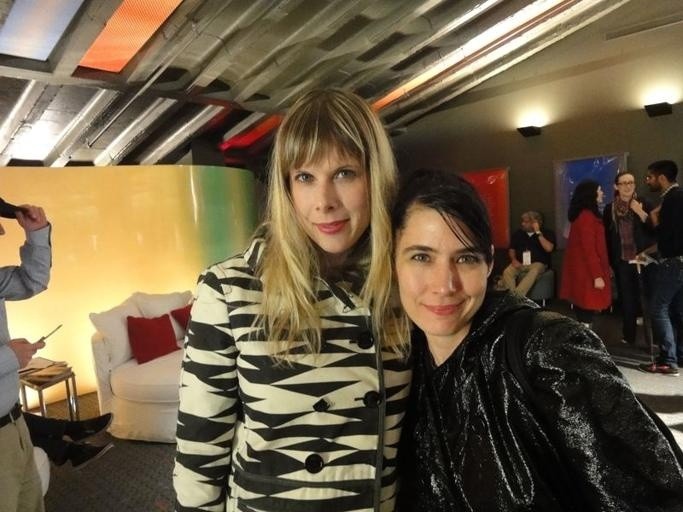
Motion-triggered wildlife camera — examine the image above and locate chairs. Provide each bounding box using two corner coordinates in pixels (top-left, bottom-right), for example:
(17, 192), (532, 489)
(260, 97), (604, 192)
(87, 326), (196, 445)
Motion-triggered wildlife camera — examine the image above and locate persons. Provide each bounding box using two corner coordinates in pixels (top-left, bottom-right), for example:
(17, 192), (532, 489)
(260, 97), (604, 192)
(0, 197), (61, 512)
(558, 179), (615, 330)
(171, 85), (410, 511)
(635, 159), (682, 377)
(19, 408), (116, 471)
(496, 202), (552, 296)
(602, 170), (654, 345)
(391, 166), (682, 512)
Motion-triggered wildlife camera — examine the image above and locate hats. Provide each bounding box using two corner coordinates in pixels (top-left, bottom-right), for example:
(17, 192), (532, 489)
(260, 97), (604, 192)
(1, 197), (29, 220)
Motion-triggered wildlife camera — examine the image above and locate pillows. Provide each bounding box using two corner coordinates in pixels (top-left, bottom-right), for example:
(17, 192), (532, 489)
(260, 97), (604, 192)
(127, 312), (181, 365)
(88, 298), (146, 368)
(132, 290), (189, 343)
(169, 303), (193, 329)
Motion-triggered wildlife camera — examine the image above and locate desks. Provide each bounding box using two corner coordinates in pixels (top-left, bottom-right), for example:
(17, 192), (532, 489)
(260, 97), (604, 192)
(17, 356), (82, 424)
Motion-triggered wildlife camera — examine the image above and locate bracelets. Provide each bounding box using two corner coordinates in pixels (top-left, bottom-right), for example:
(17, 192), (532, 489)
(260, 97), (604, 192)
(535, 230), (541, 235)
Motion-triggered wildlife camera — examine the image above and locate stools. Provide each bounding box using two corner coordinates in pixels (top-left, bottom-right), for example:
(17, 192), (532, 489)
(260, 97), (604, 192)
(516, 269), (555, 308)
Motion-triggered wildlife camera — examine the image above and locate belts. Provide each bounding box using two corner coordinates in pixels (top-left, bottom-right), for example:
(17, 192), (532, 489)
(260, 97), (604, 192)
(0, 399), (23, 428)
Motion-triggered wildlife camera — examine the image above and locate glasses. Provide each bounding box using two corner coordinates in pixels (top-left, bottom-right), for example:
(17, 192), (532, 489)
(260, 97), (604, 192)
(616, 180), (635, 185)
(646, 174), (665, 180)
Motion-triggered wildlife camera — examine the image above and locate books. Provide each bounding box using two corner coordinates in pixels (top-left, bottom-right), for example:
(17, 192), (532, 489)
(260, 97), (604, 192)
(18, 356), (71, 389)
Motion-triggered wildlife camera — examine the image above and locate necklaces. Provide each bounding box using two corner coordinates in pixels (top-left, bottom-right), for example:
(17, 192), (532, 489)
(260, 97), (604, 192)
(659, 183), (679, 199)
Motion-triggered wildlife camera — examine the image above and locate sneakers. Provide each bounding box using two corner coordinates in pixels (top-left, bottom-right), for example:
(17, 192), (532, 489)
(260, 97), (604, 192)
(71, 412), (114, 442)
(637, 362), (680, 377)
(616, 338), (633, 348)
(71, 442), (115, 472)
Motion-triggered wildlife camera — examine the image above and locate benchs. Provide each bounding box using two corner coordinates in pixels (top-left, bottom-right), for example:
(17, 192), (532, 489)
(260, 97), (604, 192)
(29, 410), (49, 497)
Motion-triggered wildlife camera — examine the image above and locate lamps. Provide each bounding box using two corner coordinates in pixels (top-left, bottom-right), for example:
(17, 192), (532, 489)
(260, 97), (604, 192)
(517, 125), (542, 140)
(642, 101), (672, 119)
